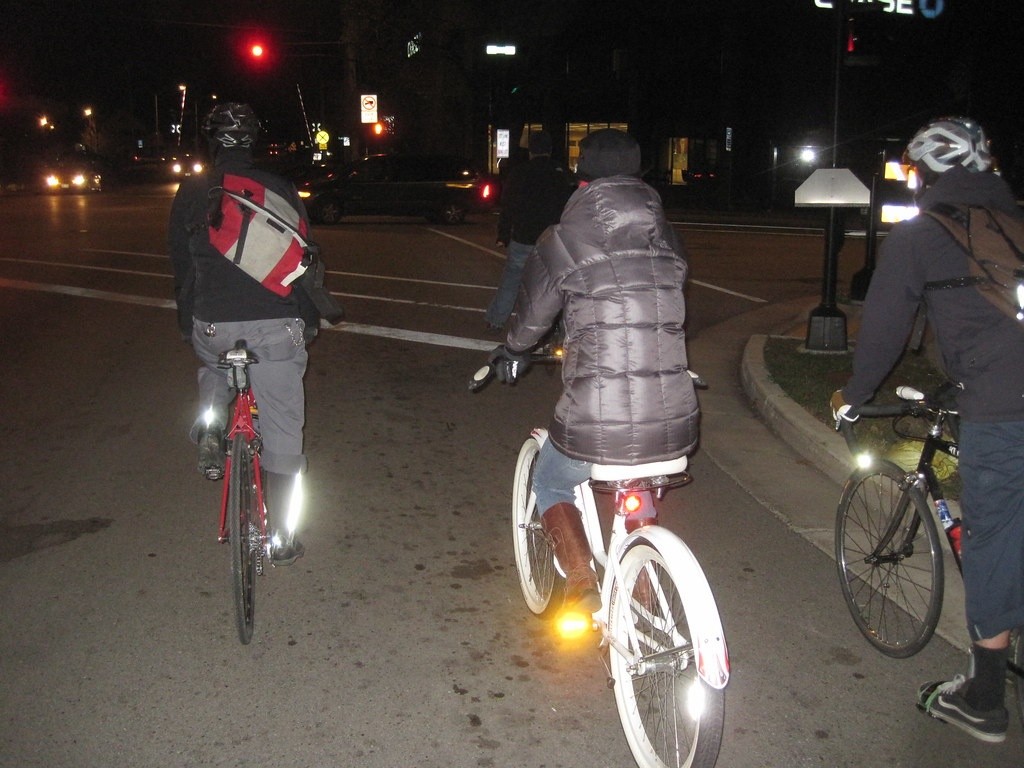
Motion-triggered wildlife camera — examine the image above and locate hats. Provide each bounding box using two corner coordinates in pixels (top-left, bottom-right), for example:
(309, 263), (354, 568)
(577, 128), (641, 181)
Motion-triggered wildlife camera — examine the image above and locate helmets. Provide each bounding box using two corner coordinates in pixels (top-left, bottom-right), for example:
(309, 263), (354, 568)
(901, 115), (995, 187)
(201, 97), (258, 148)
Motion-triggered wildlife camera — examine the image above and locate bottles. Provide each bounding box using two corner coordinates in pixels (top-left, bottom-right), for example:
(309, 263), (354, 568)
(250, 406), (260, 435)
(950, 517), (962, 561)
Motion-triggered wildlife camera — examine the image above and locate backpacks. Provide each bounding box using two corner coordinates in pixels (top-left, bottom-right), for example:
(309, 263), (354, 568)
(922, 201), (1024, 329)
(206, 154), (345, 325)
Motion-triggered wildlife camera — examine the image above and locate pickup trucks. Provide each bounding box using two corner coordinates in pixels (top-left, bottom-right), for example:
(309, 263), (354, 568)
(299, 150), (492, 227)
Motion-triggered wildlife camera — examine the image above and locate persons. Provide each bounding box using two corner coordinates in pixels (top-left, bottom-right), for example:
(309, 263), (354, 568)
(484, 131), (578, 329)
(487, 127), (701, 634)
(168, 101), (321, 566)
(828, 116), (1024, 742)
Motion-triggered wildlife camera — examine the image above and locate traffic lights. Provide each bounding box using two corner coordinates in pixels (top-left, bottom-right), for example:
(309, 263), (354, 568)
(368, 118), (391, 141)
(248, 42), (269, 62)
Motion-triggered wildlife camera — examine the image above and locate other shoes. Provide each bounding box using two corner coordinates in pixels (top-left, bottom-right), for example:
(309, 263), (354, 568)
(485, 322), (503, 337)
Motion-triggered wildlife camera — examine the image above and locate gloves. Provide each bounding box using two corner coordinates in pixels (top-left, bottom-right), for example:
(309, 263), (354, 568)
(828, 389), (861, 430)
(488, 344), (532, 383)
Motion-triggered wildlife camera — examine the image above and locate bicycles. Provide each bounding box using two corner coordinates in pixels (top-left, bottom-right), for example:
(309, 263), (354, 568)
(206, 339), (274, 643)
(834, 393), (1024, 702)
(468, 340), (737, 768)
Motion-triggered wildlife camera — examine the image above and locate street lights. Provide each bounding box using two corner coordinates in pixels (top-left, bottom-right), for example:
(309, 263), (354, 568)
(177, 85), (188, 146)
(482, 42), (516, 175)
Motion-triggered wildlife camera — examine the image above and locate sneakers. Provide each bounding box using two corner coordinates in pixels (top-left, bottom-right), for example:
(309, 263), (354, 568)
(919, 674), (1008, 743)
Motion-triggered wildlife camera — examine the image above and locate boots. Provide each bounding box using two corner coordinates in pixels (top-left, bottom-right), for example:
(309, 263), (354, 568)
(626, 516), (658, 628)
(198, 420), (227, 480)
(264, 470), (304, 566)
(541, 502), (601, 637)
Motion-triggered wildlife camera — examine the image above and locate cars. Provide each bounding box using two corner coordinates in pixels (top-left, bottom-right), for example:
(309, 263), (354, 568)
(39, 151), (104, 195)
